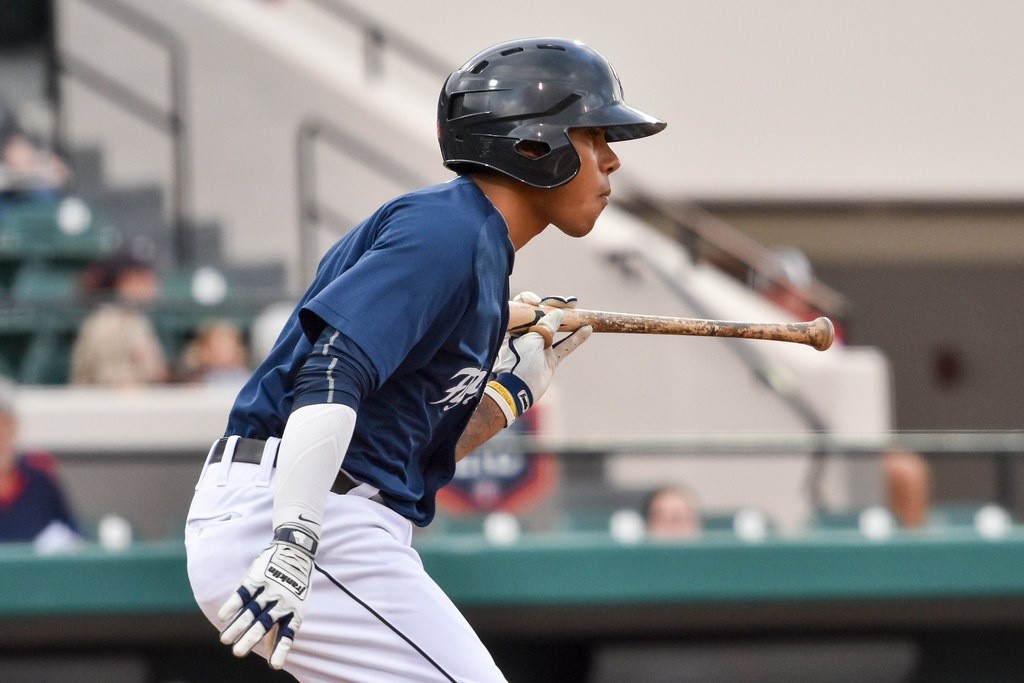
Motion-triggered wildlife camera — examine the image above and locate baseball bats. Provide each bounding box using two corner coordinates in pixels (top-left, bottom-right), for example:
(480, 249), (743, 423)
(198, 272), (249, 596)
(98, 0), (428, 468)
(505, 300), (834, 352)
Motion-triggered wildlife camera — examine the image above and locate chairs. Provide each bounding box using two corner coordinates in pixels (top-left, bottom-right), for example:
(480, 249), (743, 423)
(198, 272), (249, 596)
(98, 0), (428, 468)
(0, 198), (1004, 551)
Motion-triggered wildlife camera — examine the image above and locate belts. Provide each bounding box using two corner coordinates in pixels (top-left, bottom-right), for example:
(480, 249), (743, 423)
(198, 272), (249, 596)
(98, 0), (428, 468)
(209, 436), (361, 494)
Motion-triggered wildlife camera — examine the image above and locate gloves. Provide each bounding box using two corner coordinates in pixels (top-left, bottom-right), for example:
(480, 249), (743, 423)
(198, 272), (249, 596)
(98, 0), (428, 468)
(214, 522), (320, 670)
(484, 288), (592, 431)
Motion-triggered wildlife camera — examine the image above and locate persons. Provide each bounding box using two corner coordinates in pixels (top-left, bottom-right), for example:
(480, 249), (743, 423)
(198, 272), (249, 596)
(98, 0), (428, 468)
(0, 398), (86, 544)
(181, 36), (625, 683)
(65, 253), (259, 386)
(743, 246), (853, 349)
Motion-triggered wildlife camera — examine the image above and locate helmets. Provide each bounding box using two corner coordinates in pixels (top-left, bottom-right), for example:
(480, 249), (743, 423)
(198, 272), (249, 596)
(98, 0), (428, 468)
(435, 36), (667, 192)
